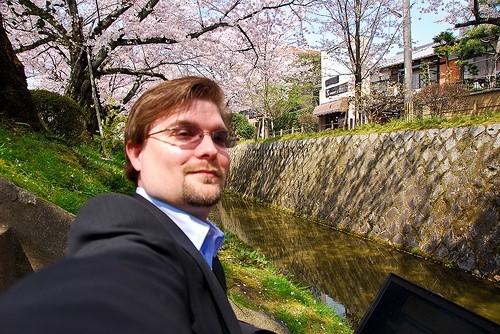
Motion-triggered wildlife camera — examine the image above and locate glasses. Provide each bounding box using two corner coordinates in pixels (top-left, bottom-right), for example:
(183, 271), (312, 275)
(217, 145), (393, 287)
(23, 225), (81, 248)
(144, 123), (240, 149)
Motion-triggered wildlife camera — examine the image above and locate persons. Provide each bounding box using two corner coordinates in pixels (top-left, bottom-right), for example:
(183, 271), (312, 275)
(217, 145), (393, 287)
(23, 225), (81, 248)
(0, 75), (277, 333)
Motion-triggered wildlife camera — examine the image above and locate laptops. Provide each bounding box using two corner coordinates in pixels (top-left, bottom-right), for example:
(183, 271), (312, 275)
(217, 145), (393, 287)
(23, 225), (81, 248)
(353, 273), (499, 334)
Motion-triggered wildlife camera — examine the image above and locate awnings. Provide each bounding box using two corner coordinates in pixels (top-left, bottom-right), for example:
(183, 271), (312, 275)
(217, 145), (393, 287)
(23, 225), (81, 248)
(312, 97), (348, 116)
